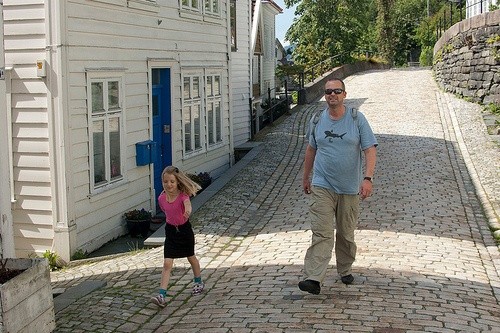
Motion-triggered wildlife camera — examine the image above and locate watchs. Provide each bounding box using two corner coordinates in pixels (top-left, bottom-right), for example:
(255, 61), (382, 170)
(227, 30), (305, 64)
(364, 177), (373, 183)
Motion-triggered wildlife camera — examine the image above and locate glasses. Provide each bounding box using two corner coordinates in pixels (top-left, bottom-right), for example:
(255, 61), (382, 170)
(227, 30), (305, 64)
(325, 88), (345, 94)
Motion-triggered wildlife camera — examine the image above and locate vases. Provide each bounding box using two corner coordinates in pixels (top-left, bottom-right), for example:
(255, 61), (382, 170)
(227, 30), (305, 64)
(199, 178), (210, 192)
(128, 219), (150, 239)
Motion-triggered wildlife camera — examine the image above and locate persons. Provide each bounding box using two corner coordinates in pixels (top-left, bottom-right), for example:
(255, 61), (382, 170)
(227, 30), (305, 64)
(298, 78), (379, 296)
(151, 166), (204, 308)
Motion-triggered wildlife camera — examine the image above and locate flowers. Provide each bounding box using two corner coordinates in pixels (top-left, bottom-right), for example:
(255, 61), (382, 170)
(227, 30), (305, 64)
(188, 170), (211, 184)
(123, 208), (152, 221)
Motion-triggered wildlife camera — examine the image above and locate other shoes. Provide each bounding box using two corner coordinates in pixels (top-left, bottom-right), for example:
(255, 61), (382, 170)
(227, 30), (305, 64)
(341, 274), (354, 285)
(298, 280), (321, 295)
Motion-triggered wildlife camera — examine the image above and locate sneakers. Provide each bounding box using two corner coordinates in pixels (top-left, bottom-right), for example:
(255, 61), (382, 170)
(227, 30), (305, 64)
(192, 282), (205, 296)
(151, 294), (166, 307)
